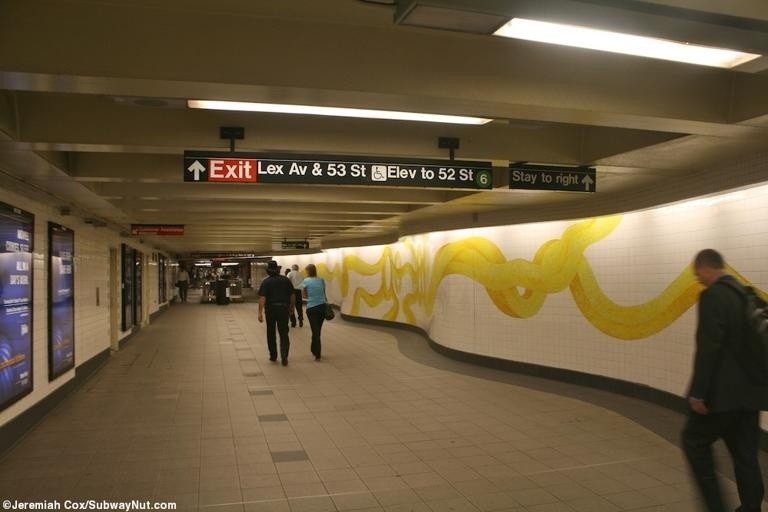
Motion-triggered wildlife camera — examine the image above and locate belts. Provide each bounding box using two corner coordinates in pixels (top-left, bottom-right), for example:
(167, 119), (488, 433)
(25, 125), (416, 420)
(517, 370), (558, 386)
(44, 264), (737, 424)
(269, 302), (288, 307)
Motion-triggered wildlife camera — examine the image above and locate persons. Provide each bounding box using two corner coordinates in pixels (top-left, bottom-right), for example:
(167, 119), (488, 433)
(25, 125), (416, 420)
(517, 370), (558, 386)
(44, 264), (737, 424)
(286, 263), (306, 327)
(300, 263), (328, 361)
(193, 267), (231, 290)
(177, 266), (191, 303)
(285, 269), (304, 320)
(257, 260), (296, 366)
(678, 249), (765, 512)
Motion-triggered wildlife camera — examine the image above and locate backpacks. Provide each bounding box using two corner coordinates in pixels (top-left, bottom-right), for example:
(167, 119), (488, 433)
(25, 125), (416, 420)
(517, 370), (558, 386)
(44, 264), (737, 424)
(716, 279), (767, 385)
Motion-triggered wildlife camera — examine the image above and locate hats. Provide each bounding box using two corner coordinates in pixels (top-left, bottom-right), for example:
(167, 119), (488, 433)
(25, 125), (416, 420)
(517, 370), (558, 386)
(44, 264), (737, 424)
(265, 260), (282, 274)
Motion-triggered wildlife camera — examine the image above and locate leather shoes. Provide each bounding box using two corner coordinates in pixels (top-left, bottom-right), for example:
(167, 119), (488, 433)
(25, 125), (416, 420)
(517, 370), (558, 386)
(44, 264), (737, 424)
(269, 356), (277, 361)
(299, 317), (303, 327)
(291, 323), (296, 327)
(281, 352), (288, 366)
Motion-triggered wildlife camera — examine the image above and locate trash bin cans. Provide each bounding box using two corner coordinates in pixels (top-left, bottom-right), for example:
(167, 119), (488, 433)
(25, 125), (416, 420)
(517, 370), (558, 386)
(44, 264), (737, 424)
(200, 285), (209, 303)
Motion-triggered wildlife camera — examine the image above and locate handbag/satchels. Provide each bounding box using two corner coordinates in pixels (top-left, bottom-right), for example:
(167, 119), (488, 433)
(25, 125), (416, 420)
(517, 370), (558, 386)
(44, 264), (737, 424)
(324, 302), (335, 320)
